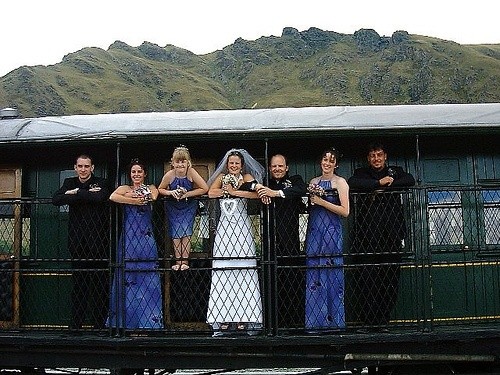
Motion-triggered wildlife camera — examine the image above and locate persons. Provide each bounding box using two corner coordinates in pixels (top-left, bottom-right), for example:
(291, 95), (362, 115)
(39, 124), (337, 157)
(306, 148), (349, 334)
(158, 147), (210, 271)
(347, 144), (415, 333)
(206, 148), (267, 337)
(52, 155), (115, 336)
(239, 154), (306, 336)
(105, 160), (165, 329)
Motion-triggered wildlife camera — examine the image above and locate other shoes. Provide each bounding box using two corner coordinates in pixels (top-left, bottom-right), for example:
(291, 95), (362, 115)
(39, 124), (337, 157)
(171, 264), (189, 271)
(357, 328), (369, 334)
(378, 328), (390, 333)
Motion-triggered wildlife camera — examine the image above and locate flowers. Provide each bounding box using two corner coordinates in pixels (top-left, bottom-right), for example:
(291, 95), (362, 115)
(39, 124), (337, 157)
(220, 174), (240, 198)
(306, 183), (327, 206)
(133, 183), (151, 205)
